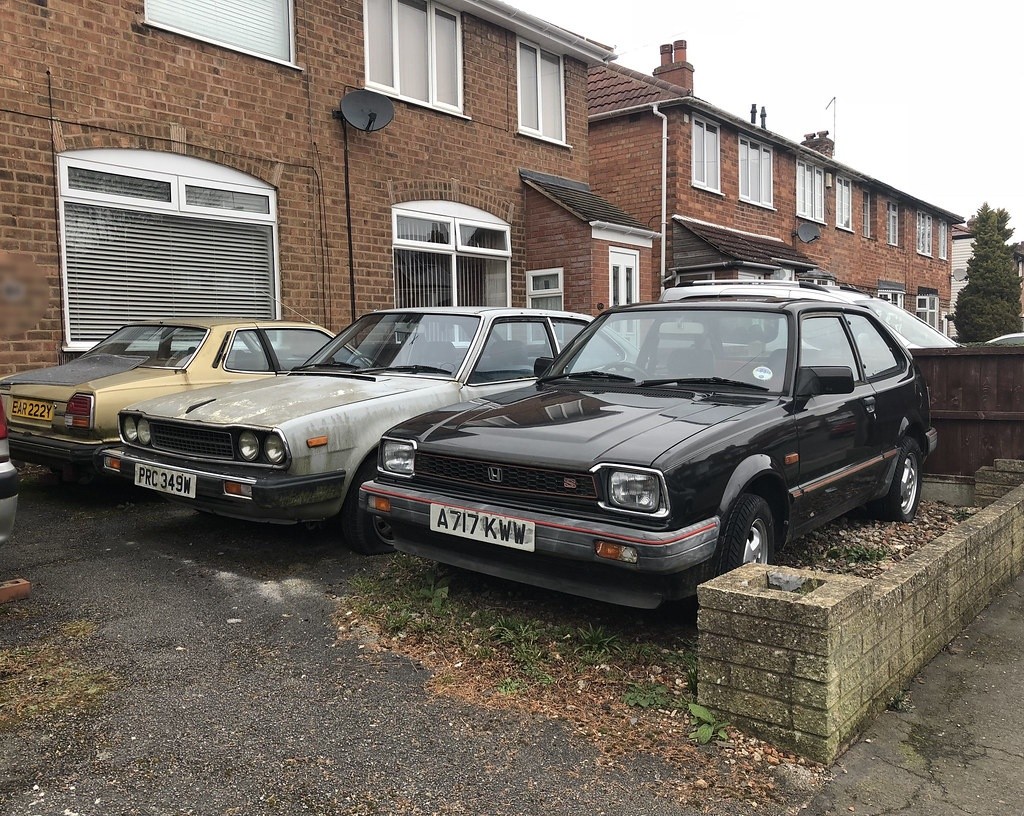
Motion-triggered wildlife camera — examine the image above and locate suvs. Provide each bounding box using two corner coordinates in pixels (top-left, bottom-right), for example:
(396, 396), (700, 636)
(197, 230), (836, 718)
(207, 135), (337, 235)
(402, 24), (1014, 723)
(658, 279), (966, 360)
(359, 297), (938, 610)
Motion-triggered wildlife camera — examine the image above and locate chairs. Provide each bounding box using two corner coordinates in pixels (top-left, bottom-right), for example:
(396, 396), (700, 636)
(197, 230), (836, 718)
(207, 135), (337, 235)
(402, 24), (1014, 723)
(481, 340), (534, 371)
(766, 349), (822, 382)
(420, 341), (460, 373)
(665, 347), (714, 376)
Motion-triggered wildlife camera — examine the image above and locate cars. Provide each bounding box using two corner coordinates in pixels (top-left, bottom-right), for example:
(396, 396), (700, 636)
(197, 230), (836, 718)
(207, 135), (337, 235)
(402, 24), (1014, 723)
(92, 306), (642, 555)
(0, 317), (379, 545)
